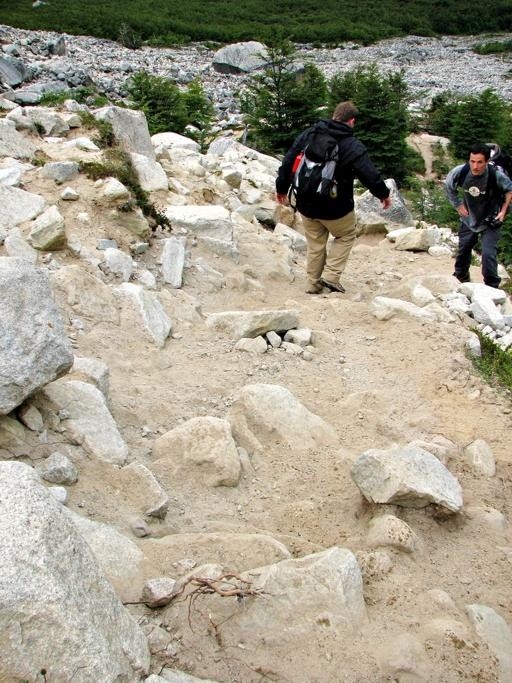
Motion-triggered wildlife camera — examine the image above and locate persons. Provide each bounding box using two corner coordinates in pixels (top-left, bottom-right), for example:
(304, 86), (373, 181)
(445, 141), (511, 288)
(275, 100), (390, 295)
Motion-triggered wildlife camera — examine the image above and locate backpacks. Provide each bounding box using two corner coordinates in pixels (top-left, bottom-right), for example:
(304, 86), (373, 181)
(289, 126), (352, 204)
(458, 142), (511, 205)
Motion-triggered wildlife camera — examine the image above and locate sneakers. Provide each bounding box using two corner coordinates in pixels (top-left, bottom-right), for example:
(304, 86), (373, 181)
(305, 277), (346, 292)
(452, 270), (470, 282)
